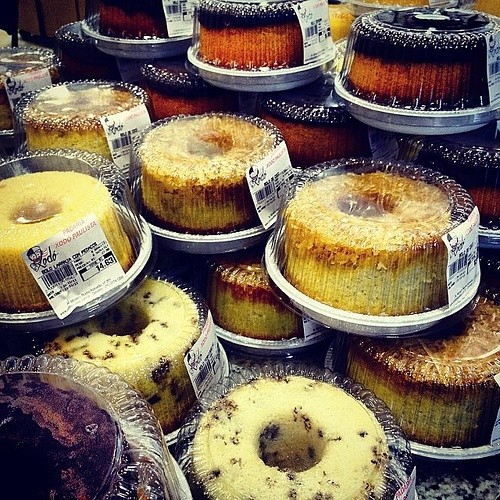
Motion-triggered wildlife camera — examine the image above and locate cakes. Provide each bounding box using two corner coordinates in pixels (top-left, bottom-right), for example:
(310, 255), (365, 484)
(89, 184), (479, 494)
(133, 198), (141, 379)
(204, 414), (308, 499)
(0, 0), (500, 500)
